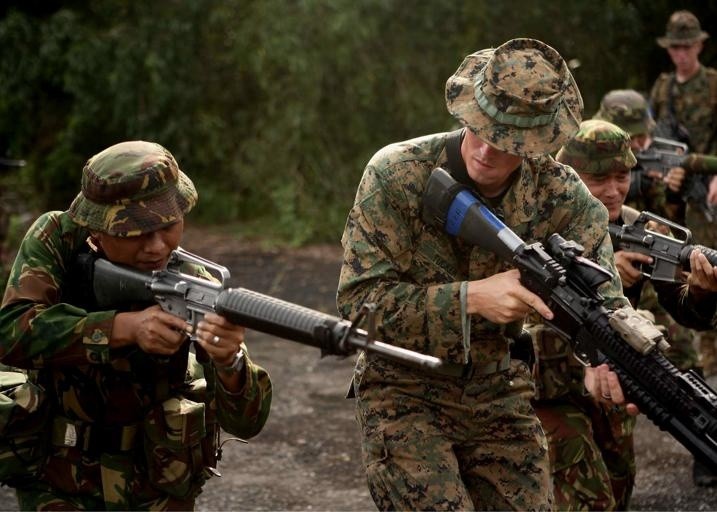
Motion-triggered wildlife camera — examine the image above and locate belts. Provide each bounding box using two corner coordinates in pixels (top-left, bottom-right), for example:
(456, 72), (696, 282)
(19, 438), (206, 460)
(49, 416), (138, 451)
(376, 351), (511, 377)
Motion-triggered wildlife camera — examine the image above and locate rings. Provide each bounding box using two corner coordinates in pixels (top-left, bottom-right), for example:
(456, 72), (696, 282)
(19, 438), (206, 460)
(601, 392), (612, 400)
(212, 336), (220, 344)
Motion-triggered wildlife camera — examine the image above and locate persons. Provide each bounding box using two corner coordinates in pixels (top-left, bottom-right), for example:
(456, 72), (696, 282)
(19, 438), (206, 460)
(334, 36), (641, 512)
(648, 11), (717, 378)
(0, 140), (273, 512)
(592, 89), (705, 379)
(521, 118), (717, 512)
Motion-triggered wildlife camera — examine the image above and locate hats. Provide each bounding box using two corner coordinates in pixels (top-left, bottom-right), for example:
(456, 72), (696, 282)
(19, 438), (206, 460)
(69, 141), (198, 237)
(555, 120), (637, 172)
(592, 89), (656, 135)
(444, 39), (584, 158)
(657, 12), (707, 47)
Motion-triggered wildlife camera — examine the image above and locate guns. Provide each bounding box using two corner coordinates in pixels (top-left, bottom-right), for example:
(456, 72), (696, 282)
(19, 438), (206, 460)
(91, 248), (445, 377)
(609, 210), (716, 285)
(631, 136), (717, 181)
(418, 166), (717, 478)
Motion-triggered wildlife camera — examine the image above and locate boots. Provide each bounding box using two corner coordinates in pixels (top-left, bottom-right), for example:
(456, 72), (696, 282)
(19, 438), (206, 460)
(678, 367), (717, 487)
